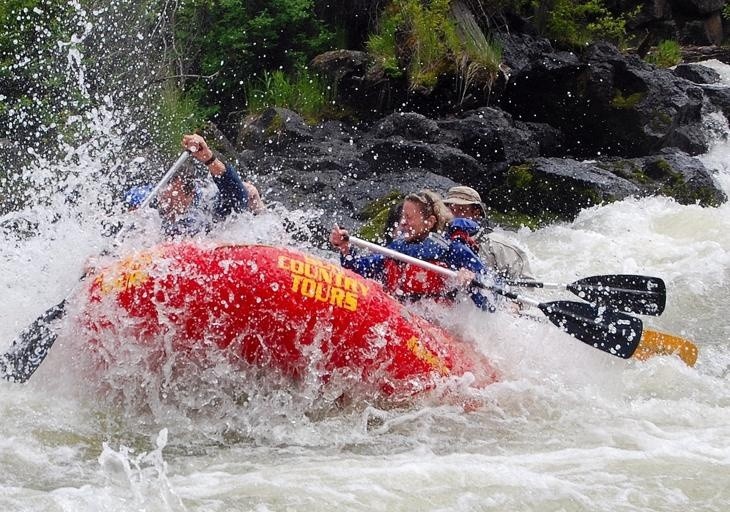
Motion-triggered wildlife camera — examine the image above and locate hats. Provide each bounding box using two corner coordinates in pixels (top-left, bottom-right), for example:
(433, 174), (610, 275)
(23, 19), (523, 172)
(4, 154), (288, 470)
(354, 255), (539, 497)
(442, 186), (486, 218)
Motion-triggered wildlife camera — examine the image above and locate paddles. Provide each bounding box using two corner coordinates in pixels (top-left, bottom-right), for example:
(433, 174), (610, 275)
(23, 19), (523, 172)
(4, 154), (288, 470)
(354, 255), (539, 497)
(1, 147), (197, 383)
(629, 329), (698, 369)
(496, 274), (666, 316)
(342, 234), (642, 358)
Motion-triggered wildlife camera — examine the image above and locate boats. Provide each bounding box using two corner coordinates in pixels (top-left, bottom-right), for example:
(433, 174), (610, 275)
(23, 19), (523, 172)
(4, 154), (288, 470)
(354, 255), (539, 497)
(81, 237), (504, 417)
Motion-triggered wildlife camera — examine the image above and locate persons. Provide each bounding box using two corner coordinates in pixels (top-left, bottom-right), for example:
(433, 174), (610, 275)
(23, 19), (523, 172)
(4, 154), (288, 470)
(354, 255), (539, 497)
(329, 183), (539, 312)
(107, 130), (313, 254)
